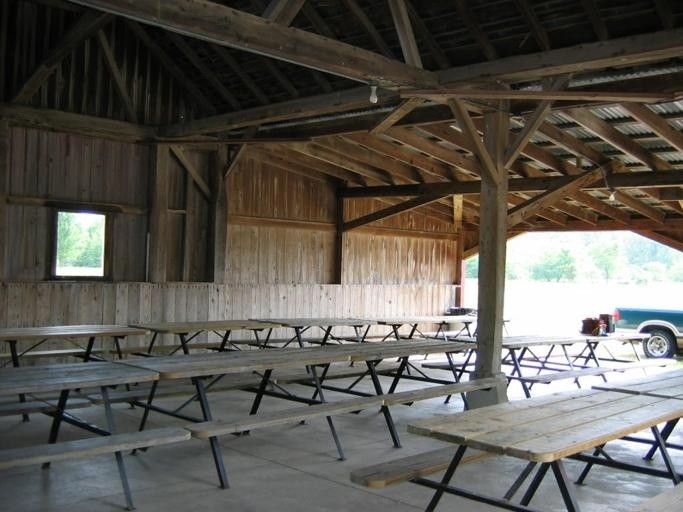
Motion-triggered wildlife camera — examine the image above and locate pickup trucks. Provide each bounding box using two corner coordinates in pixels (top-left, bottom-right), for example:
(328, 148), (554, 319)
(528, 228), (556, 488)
(612, 307), (683, 358)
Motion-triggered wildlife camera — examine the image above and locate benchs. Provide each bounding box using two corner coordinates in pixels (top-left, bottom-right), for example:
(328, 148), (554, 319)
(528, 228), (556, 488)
(1, 428), (192, 511)
(508, 359), (636, 384)
(185, 374), (511, 489)
(231, 336), (327, 349)
(422, 360), (476, 372)
(1, 398), (92, 416)
(122, 340), (236, 354)
(350, 444), (505, 491)
(90, 362), (413, 407)
(0, 427), (194, 470)
(187, 376), (497, 440)
(0, 348), (107, 359)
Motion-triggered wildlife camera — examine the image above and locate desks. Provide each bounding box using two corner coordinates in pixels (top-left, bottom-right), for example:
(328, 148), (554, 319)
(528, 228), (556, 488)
(374, 313), (480, 377)
(110, 336), (479, 490)
(592, 368), (683, 461)
(407, 388), (682, 512)
(443, 334), (651, 399)
(125, 317), (286, 386)
(0, 363), (161, 512)
(248, 314), (379, 374)
(0, 323), (149, 421)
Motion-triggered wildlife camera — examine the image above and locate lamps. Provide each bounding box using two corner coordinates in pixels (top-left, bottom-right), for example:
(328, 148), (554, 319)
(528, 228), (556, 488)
(369, 83), (380, 105)
